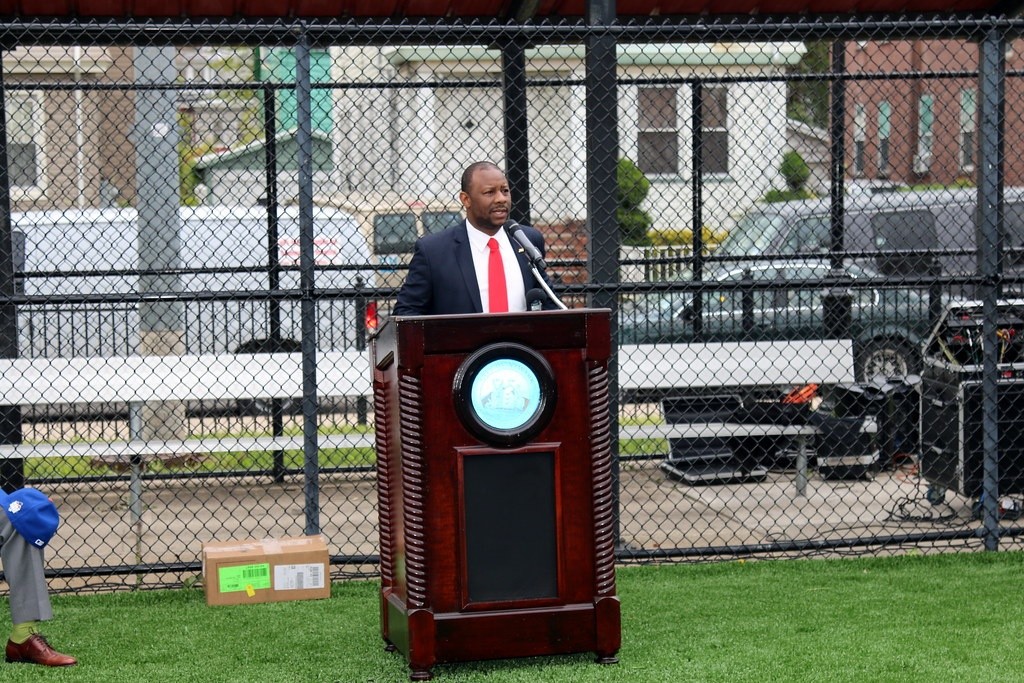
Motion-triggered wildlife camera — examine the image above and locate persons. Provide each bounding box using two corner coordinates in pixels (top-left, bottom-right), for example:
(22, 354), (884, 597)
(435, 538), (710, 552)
(391, 160), (559, 317)
(0, 486), (79, 668)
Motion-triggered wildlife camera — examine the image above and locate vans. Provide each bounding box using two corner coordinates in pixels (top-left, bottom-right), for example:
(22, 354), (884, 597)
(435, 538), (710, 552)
(655, 185), (1023, 297)
(12, 206), (378, 417)
(290, 194), (463, 321)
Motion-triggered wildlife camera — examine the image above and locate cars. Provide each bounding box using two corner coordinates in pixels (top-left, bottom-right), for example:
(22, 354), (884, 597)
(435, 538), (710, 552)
(619, 260), (967, 384)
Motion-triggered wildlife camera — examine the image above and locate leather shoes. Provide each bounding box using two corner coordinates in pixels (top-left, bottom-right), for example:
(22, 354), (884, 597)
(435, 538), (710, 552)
(5, 626), (78, 667)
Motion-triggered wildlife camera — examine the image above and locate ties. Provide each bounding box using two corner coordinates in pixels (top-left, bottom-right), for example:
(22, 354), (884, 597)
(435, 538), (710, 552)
(488, 238), (508, 313)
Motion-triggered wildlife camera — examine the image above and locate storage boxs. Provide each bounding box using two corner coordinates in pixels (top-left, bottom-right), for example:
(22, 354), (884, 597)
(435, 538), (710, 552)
(201, 533), (330, 607)
(915, 374), (1024, 523)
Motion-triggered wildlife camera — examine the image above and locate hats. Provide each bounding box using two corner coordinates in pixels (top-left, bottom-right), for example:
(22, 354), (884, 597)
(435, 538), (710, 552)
(0, 486), (59, 549)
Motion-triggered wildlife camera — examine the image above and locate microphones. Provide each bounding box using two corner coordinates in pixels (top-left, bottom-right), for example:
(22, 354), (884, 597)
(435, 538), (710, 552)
(504, 219), (547, 269)
(526, 288), (547, 311)
